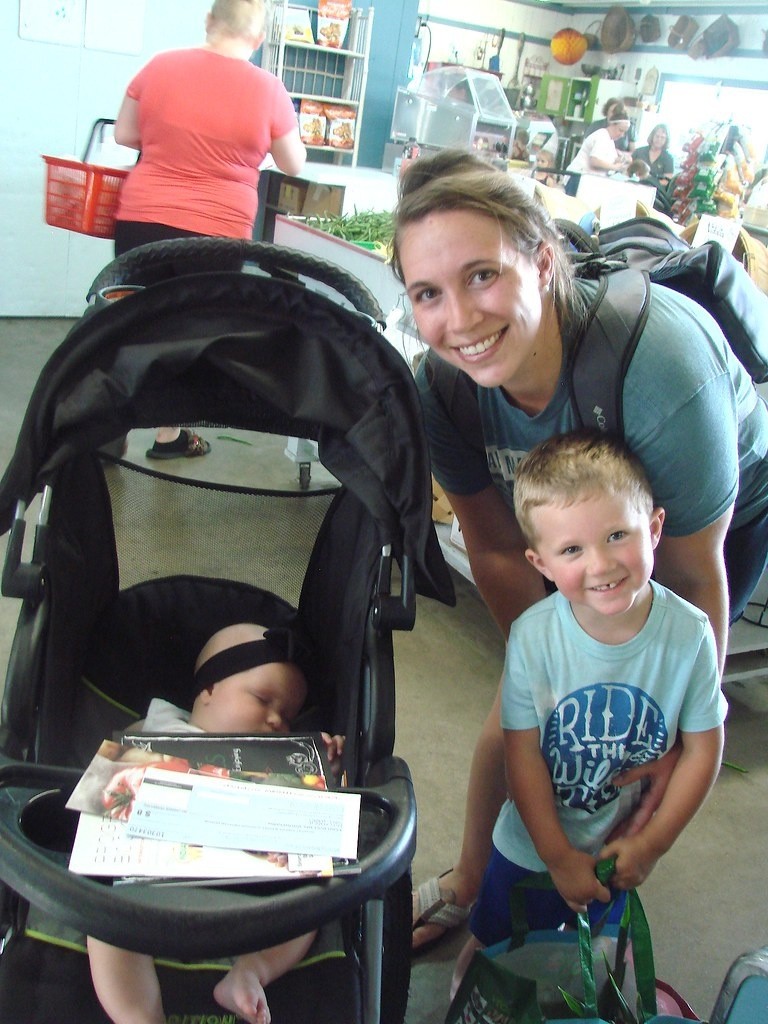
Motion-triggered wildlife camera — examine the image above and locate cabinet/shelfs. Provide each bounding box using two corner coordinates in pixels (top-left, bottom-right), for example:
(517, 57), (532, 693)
(242, 155), (395, 265)
(264, 0), (374, 166)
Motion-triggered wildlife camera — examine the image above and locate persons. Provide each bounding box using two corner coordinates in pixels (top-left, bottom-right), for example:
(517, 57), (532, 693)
(583, 99), (621, 141)
(107, 0), (309, 461)
(624, 160), (652, 182)
(633, 124), (675, 189)
(565, 115), (633, 176)
(513, 131), (530, 163)
(528, 151), (556, 187)
(443, 430), (731, 1014)
(64, 623), (350, 1024)
(387, 146), (767, 699)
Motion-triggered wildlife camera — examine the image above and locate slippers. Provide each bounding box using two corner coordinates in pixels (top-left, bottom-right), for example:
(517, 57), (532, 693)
(408, 868), (477, 958)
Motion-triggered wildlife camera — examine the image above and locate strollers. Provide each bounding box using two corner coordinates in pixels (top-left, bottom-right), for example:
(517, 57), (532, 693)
(0, 233), (455, 1024)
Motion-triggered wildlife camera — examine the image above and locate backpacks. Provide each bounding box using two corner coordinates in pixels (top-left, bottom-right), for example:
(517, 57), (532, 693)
(425, 213), (768, 456)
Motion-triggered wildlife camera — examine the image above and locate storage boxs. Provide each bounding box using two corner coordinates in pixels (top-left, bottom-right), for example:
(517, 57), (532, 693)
(278, 177), (305, 214)
(303, 181), (343, 219)
(431, 470), (454, 523)
(450, 513), (466, 551)
(539, 74), (636, 124)
